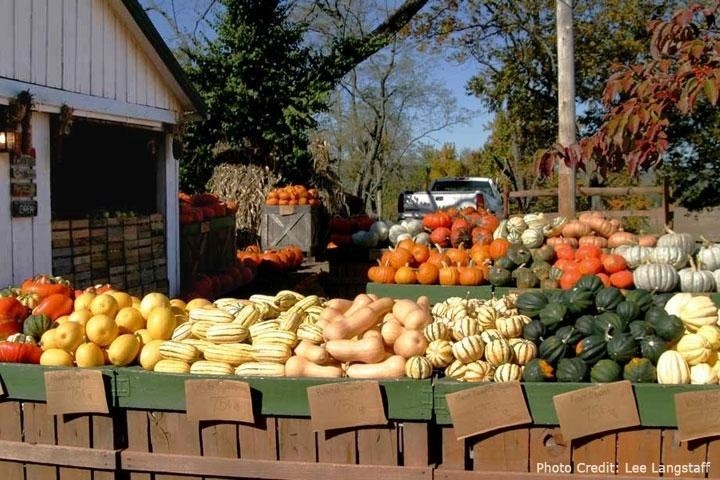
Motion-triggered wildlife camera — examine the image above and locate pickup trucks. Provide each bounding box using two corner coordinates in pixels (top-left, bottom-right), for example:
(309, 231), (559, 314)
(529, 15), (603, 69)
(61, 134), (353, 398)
(398, 174), (506, 224)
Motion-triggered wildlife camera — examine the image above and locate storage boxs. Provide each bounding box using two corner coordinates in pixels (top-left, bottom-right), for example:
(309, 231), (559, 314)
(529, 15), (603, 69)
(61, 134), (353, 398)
(179, 216), (236, 288)
(261, 204), (320, 258)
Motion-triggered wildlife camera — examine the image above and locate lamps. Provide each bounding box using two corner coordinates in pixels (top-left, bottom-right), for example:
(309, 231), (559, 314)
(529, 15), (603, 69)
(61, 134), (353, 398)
(0, 120), (17, 153)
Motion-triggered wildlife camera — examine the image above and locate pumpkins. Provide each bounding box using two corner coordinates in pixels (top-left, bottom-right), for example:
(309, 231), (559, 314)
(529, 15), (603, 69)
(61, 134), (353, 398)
(0, 184), (720, 384)
